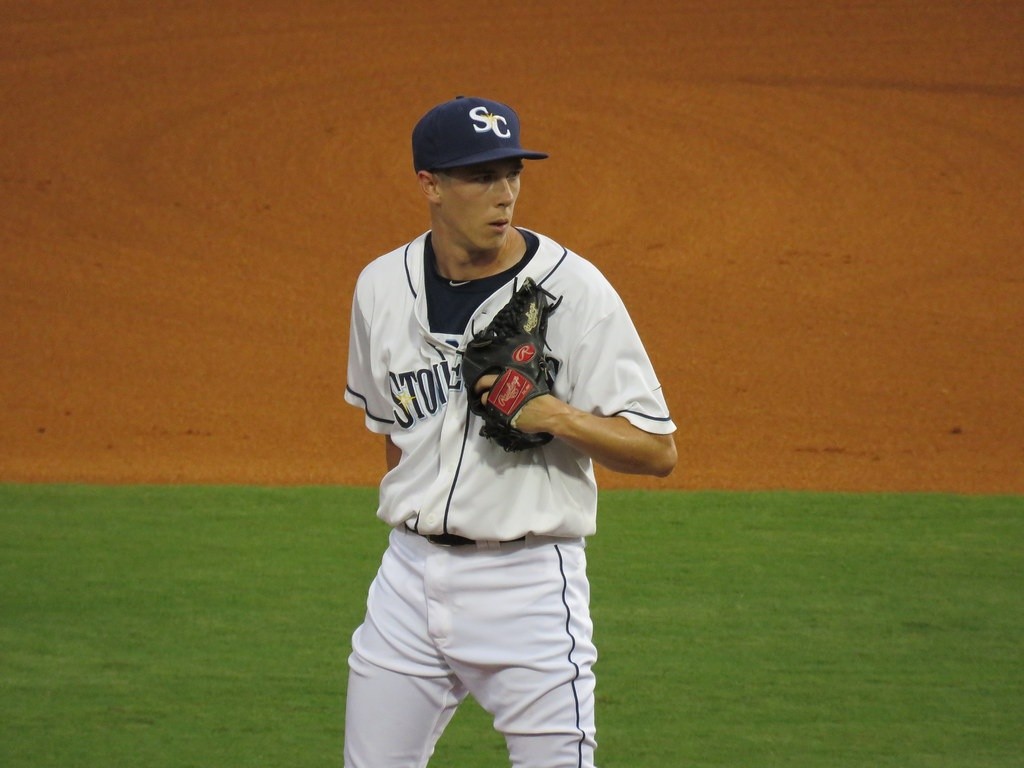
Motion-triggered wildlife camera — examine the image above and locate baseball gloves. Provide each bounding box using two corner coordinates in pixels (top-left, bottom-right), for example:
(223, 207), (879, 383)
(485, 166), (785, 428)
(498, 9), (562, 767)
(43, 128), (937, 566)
(461, 277), (560, 455)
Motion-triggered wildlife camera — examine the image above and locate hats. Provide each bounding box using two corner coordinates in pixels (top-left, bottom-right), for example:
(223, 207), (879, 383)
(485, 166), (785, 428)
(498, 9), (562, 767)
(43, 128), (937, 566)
(412, 96), (549, 174)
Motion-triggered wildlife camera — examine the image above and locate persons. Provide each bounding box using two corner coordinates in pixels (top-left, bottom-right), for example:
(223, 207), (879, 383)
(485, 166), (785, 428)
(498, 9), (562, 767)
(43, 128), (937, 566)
(345, 91), (677, 768)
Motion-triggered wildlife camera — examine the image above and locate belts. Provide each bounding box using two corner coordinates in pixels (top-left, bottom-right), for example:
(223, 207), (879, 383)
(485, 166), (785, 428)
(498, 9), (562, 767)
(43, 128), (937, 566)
(405, 523), (526, 547)
